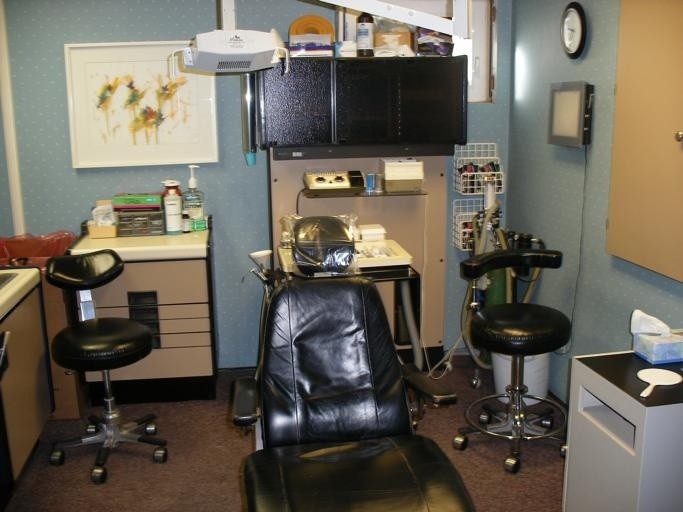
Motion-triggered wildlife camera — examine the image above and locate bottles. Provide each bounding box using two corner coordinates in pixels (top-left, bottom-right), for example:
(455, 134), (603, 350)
(161, 178), (182, 232)
(181, 211), (191, 233)
(364, 174), (384, 193)
(338, 212), (360, 241)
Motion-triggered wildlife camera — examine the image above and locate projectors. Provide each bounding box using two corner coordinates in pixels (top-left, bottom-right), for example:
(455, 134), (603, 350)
(182, 29), (276, 73)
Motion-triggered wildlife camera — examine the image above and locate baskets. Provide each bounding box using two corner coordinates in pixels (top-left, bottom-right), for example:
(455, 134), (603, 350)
(453, 142), (506, 196)
(451, 199), (500, 253)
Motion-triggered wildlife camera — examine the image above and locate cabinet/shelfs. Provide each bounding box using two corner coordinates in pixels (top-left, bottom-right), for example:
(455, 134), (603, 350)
(398, 53), (469, 145)
(329, 53), (401, 144)
(254, 55), (335, 147)
(0, 285), (56, 484)
(22, 258), (85, 423)
(63, 215), (218, 404)
(560, 349), (683, 511)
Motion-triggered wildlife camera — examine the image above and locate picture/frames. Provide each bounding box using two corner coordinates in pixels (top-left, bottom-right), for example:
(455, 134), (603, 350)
(61, 39), (222, 171)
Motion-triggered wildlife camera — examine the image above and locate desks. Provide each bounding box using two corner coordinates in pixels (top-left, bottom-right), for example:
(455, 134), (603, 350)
(277, 248), (424, 372)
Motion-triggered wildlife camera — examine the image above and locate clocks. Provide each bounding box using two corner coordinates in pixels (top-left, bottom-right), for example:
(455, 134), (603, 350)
(557, 2), (587, 57)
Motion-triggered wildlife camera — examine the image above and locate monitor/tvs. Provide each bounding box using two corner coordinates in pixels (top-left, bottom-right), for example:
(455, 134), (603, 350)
(547, 81), (595, 149)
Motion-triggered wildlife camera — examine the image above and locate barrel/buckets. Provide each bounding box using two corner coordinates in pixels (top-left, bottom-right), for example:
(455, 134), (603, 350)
(493, 352), (550, 407)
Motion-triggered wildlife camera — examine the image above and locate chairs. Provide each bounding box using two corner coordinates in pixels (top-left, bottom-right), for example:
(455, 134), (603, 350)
(449, 245), (571, 473)
(226, 275), (479, 512)
(40, 248), (177, 486)
